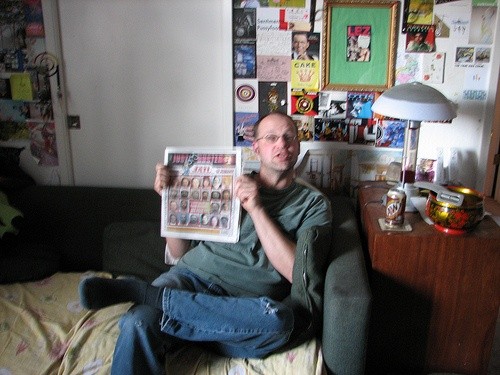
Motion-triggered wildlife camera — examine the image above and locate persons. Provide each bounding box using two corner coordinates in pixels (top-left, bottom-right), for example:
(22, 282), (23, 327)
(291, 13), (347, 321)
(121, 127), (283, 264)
(407, 29), (432, 51)
(80, 111), (332, 375)
(292, 32), (317, 60)
(169, 177), (231, 228)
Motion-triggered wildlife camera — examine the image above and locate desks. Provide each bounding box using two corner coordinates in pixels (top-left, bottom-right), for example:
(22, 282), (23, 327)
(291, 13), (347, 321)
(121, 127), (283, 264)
(359, 181), (500, 375)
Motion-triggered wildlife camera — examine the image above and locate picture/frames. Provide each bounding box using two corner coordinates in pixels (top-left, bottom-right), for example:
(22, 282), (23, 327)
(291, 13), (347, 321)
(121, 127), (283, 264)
(320, 0), (399, 92)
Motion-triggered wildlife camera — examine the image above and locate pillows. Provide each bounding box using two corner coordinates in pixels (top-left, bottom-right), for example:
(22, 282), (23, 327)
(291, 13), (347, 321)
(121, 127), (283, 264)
(288, 226), (331, 348)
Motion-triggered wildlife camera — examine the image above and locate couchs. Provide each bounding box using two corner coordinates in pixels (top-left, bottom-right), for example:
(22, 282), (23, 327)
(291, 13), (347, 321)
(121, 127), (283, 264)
(0, 184), (367, 375)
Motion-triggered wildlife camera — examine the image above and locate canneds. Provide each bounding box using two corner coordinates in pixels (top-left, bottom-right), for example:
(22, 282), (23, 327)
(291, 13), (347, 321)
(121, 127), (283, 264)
(384, 189), (406, 228)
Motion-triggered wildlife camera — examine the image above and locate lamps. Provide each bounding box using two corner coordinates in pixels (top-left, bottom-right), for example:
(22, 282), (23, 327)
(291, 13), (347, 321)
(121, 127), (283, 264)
(371, 82), (455, 212)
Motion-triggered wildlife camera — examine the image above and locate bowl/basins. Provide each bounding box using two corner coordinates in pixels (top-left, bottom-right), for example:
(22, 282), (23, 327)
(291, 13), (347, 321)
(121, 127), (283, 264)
(424, 185), (485, 236)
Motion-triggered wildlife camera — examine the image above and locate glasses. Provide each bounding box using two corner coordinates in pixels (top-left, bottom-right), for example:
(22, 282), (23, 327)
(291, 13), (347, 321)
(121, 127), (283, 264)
(256, 133), (300, 145)
